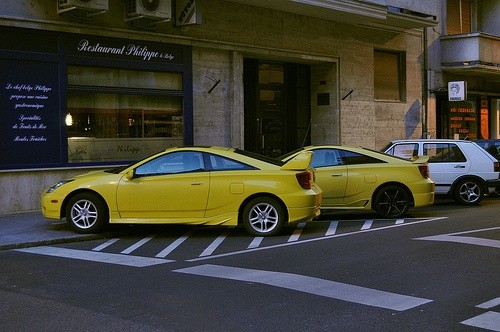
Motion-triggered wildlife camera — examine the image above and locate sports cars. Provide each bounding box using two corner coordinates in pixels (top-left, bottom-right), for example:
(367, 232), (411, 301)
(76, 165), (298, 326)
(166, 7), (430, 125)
(42, 146), (322, 237)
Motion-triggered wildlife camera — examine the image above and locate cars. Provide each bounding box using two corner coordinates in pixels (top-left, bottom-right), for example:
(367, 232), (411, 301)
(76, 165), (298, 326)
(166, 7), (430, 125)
(381, 138), (500, 205)
(277, 144), (434, 219)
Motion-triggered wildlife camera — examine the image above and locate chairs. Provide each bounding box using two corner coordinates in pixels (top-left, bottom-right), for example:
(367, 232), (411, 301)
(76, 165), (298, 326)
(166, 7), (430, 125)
(325, 152), (335, 164)
(183, 155), (200, 170)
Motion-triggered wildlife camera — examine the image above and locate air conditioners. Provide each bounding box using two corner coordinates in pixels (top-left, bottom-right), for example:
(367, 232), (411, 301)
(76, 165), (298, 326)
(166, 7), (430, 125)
(124, 0), (171, 25)
(56, 0), (108, 17)
